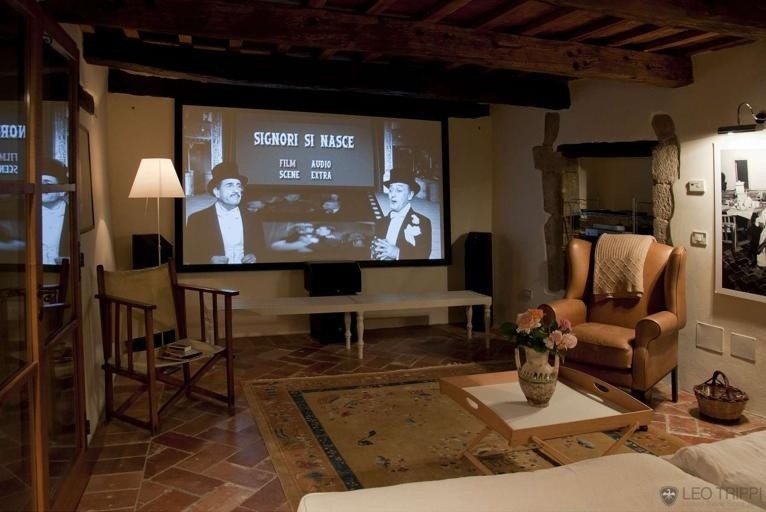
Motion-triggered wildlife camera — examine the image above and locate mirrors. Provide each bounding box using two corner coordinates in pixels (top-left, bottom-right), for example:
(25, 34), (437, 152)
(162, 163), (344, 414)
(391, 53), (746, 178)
(0, 0), (82, 512)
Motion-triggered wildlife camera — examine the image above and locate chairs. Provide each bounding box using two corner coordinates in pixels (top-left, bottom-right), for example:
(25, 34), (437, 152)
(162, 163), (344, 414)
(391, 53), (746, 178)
(94, 260), (239, 436)
(537, 234), (687, 431)
(0, 259), (73, 437)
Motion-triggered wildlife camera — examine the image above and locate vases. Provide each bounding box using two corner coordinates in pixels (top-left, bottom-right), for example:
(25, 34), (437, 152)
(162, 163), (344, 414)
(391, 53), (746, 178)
(515, 342), (560, 407)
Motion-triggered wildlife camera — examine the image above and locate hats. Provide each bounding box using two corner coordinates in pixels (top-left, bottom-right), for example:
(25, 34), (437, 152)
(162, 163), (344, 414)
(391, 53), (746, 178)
(41, 160), (67, 184)
(207, 162), (248, 197)
(384, 169), (420, 195)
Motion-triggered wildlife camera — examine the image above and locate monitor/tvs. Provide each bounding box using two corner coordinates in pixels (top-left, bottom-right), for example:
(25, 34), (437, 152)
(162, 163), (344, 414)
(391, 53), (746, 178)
(174, 95), (454, 274)
(0, 90), (76, 274)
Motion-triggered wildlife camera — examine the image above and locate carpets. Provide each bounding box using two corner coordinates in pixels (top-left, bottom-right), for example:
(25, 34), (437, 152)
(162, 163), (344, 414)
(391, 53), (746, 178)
(240, 357), (692, 511)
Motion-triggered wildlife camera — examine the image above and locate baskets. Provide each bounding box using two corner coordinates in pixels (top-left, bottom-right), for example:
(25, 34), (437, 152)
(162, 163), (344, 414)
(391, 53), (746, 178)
(693, 371), (749, 419)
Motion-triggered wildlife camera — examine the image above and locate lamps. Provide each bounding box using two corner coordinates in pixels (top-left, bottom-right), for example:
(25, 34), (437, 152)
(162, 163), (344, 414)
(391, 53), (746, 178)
(128, 158), (187, 263)
(717, 103), (765, 135)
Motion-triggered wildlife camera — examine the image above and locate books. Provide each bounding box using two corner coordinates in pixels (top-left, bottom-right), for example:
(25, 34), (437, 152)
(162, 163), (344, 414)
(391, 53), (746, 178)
(153, 342), (203, 361)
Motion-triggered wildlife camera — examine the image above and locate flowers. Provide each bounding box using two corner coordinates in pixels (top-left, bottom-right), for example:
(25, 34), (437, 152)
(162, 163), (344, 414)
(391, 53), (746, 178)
(500, 307), (578, 365)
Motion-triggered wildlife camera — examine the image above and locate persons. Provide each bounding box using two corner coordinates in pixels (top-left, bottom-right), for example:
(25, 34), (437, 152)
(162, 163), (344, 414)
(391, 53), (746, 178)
(39, 156), (69, 264)
(364, 165), (433, 260)
(184, 162), (269, 268)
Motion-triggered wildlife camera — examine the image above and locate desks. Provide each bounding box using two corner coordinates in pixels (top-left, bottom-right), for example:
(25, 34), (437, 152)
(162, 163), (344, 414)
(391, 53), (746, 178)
(439, 365), (654, 478)
(202, 290), (492, 358)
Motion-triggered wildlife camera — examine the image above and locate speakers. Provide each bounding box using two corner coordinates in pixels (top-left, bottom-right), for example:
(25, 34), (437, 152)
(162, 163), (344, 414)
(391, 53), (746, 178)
(304, 263), (363, 347)
(464, 232), (494, 331)
(131, 234), (174, 352)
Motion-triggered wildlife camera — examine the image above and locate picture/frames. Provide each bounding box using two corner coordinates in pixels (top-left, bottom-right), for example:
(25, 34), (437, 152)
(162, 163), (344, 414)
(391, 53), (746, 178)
(713, 136), (766, 302)
